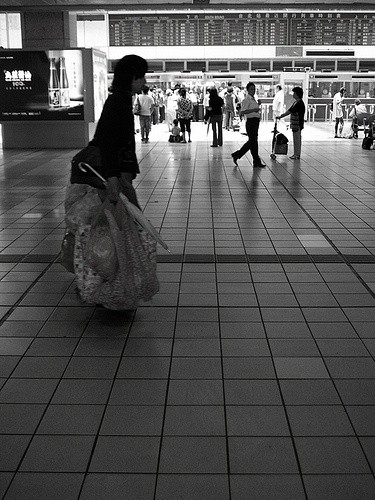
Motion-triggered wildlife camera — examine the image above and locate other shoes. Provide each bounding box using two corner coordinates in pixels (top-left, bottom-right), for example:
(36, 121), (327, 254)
(108, 309), (134, 320)
(291, 156), (300, 159)
(210, 144), (218, 147)
(254, 162), (266, 167)
(232, 153), (239, 166)
(218, 143), (222, 146)
(289, 155), (294, 158)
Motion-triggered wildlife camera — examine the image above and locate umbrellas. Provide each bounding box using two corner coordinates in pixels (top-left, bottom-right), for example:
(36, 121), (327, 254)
(76, 158), (169, 252)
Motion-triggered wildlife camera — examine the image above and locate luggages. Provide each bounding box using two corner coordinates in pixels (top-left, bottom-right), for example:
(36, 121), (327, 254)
(270, 118), (288, 160)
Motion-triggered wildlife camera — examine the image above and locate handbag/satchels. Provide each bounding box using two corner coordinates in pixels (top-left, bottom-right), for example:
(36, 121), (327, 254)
(59, 227), (75, 273)
(134, 96), (140, 114)
(362, 137), (373, 149)
(276, 133), (288, 144)
(84, 196), (122, 281)
(69, 145), (102, 185)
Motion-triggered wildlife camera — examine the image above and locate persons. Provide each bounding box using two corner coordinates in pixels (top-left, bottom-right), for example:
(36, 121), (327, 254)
(230, 83), (269, 169)
(271, 84), (289, 134)
(223, 86), (247, 131)
(125, 79), (165, 144)
(203, 88), (225, 149)
(272, 86), (305, 162)
(59, 55), (160, 318)
(362, 119), (374, 151)
(328, 87), (350, 140)
(348, 99), (367, 129)
(168, 85), (203, 145)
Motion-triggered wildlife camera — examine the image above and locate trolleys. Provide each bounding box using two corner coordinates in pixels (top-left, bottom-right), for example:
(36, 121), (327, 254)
(269, 117), (289, 160)
